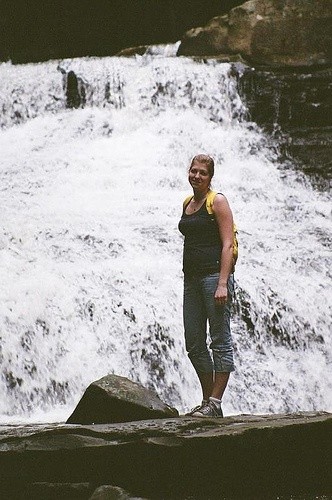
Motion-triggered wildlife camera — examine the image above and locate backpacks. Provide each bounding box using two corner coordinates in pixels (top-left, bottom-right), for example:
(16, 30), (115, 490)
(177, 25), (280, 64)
(183, 190), (239, 273)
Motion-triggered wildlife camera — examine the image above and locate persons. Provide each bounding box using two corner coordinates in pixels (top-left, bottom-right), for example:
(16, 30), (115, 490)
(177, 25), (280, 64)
(178, 154), (235, 420)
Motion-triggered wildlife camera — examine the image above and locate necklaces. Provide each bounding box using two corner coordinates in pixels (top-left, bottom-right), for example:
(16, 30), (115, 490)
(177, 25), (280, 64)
(193, 187), (209, 211)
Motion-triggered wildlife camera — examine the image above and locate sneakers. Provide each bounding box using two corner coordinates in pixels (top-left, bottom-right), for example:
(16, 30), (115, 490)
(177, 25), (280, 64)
(184, 400), (223, 417)
